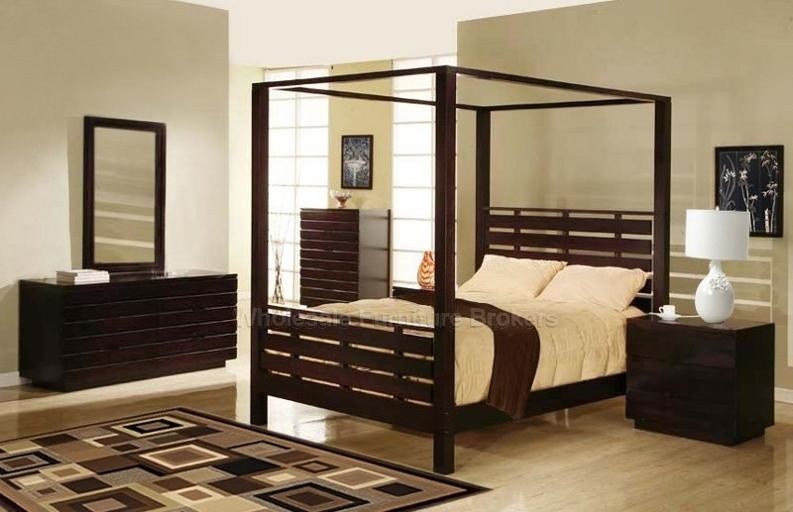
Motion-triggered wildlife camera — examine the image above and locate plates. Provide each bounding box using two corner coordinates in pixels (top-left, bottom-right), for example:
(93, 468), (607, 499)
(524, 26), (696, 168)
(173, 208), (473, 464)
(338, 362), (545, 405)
(657, 314), (681, 321)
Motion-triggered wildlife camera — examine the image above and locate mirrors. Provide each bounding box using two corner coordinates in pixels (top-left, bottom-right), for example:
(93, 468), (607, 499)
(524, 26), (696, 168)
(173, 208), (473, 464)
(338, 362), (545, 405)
(82, 115), (167, 280)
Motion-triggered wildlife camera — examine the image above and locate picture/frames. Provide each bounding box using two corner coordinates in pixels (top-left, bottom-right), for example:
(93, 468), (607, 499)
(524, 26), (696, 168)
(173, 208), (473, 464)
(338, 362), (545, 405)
(715, 145), (784, 237)
(341, 135), (373, 189)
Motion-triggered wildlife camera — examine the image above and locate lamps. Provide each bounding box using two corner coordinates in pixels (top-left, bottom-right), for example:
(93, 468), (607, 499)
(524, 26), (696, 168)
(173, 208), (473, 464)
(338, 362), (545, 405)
(685, 209), (750, 324)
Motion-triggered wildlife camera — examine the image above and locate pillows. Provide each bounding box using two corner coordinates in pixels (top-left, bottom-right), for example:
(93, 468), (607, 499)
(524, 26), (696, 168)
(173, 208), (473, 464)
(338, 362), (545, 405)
(536, 263), (648, 312)
(460, 253), (567, 298)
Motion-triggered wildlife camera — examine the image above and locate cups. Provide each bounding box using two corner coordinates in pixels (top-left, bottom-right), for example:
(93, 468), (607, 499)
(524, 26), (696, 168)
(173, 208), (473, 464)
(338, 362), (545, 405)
(658, 305), (675, 316)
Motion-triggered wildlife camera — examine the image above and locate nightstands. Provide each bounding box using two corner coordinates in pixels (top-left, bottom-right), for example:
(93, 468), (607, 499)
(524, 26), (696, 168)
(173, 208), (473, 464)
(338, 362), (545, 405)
(625, 314), (775, 447)
(392, 284), (435, 308)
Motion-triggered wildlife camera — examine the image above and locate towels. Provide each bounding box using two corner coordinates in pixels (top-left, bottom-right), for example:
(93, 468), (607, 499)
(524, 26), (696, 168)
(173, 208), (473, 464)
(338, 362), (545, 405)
(55, 268), (112, 284)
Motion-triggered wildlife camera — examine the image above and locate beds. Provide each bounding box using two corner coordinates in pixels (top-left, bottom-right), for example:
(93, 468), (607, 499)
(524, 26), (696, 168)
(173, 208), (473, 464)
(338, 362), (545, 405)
(251, 65), (671, 474)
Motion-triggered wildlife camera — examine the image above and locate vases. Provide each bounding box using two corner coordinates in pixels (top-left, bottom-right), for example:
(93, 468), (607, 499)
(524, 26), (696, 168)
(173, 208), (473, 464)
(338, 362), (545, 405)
(416, 250), (436, 291)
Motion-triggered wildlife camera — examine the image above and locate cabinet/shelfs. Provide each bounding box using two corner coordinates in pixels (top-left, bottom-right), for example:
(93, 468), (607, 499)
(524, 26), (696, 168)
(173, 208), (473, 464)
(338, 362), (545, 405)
(17, 265), (238, 395)
(298, 206), (391, 308)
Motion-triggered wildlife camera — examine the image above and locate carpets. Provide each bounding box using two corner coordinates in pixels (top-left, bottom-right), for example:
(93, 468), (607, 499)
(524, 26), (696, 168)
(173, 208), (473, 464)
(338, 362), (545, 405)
(0, 402), (491, 512)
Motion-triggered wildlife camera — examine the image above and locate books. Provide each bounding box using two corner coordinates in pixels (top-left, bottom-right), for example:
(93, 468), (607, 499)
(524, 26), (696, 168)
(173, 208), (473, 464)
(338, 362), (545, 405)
(57, 268), (110, 285)
